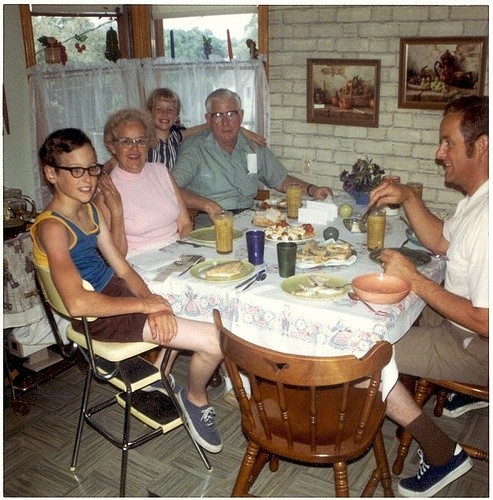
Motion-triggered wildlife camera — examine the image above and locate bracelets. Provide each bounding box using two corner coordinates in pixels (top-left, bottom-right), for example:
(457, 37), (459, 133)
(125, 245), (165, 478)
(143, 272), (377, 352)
(307, 184), (315, 196)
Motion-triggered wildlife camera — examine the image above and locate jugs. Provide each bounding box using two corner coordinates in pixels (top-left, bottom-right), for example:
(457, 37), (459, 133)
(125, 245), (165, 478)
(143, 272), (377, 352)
(3, 186), (36, 227)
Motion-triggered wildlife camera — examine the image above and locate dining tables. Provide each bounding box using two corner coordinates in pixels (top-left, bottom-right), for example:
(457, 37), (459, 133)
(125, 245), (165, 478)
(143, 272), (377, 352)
(140, 199), (447, 406)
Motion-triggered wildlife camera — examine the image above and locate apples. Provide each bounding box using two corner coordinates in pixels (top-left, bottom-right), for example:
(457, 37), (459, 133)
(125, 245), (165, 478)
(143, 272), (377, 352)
(332, 80), (374, 110)
(339, 203), (353, 219)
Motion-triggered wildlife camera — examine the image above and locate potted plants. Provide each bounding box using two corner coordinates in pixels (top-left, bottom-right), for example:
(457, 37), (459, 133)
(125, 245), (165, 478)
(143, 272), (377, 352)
(341, 158), (383, 206)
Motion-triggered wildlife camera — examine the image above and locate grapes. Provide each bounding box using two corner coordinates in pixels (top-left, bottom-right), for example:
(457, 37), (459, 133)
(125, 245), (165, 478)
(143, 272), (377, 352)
(410, 75), (450, 94)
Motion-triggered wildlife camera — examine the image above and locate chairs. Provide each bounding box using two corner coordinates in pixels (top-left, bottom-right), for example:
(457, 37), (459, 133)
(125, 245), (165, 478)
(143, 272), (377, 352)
(212, 309), (396, 498)
(31, 265), (213, 498)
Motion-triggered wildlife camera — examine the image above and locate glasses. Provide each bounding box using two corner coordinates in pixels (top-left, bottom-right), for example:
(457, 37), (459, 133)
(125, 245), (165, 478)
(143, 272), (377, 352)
(211, 110), (239, 120)
(57, 163), (104, 178)
(113, 137), (150, 150)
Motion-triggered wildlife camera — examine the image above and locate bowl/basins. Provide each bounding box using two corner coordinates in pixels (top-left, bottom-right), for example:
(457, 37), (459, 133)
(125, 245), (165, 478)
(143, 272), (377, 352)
(351, 273), (412, 304)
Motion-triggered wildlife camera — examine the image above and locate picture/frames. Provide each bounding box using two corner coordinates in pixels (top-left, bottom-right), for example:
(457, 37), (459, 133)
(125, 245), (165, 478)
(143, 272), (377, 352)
(399, 36), (488, 109)
(305, 58), (378, 127)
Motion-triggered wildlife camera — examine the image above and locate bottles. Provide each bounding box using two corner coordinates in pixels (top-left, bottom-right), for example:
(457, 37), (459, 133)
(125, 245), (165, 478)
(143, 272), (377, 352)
(105, 26), (121, 63)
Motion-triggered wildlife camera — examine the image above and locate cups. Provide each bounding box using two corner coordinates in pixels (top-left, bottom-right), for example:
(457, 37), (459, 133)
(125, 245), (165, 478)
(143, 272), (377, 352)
(277, 242), (297, 278)
(246, 230), (265, 266)
(285, 184), (302, 219)
(44, 47), (61, 63)
(214, 210), (234, 254)
(404, 182), (424, 202)
(383, 174), (401, 216)
(367, 208), (387, 252)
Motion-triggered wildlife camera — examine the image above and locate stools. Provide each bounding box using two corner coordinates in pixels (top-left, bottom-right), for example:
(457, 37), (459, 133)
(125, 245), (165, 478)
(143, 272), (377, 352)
(392, 376), (489, 475)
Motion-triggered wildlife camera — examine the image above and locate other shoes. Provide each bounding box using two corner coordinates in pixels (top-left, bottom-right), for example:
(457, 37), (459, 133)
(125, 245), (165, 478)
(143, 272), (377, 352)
(224, 372), (252, 401)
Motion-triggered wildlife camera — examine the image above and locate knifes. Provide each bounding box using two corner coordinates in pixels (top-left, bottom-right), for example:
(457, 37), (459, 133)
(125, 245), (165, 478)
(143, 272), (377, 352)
(235, 269), (265, 289)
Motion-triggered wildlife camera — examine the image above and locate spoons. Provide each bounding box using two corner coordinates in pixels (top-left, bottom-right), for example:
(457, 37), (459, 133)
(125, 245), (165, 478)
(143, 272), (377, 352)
(347, 291), (376, 313)
(242, 273), (267, 292)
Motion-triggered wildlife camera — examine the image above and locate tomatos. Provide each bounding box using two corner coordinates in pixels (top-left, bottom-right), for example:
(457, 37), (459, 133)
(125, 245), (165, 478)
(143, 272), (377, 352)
(261, 202), (271, 209)
(303, 223), (314, 233)
(276, 220), (289, 227)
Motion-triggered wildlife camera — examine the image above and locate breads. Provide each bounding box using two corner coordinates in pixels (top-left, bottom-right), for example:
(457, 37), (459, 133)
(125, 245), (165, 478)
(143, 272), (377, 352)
(298, 239), (353, 264)
(205, 261), (243, 276)
(253, 208), (279, 227)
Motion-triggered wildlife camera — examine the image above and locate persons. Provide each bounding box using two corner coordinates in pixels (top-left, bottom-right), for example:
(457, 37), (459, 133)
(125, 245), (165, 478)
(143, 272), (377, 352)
(369, 95), (490, 498)
(104, 88), (267, 174)
(30, 128), (225, 454)
(91, 109), (252, 397)
(172, 90), (333, 231)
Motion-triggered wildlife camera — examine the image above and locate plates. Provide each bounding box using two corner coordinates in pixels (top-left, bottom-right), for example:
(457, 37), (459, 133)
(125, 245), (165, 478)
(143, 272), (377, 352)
(189, 226), (244, 243)
(193, 258), (254, 281)
(368, 245), (431, 270)
(251, 218), (286, 228)
(261, 225), (320, 245)
(280, 272), (350, 299)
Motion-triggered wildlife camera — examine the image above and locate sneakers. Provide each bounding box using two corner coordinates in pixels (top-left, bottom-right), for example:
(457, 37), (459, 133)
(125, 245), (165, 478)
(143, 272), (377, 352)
(397, 441), (474, 497)
(441, 387), (489, 419)
(142, 372), (177, 393)
(179, 386), (224, 453)
